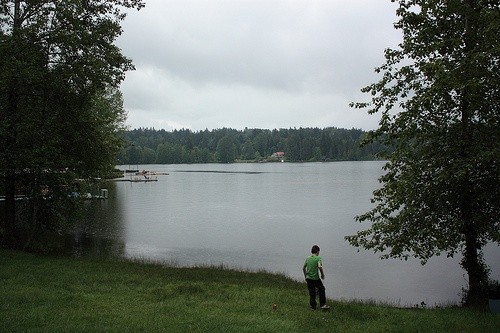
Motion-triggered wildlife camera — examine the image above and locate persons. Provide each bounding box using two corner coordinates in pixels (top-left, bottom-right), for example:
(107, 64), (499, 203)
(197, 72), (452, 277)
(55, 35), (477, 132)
(303, 245), (330, 309)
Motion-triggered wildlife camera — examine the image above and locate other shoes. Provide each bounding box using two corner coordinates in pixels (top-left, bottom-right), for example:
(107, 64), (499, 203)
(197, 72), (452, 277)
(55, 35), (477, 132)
(311, 306), (316, 311)
(320, 305), (330, 308)
